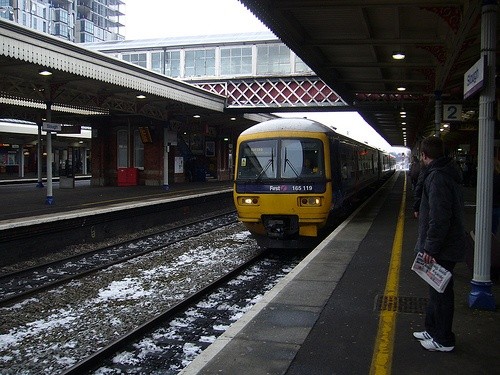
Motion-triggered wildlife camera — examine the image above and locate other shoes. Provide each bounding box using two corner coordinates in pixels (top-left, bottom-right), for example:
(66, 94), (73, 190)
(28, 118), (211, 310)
(412, 330), (455, 353)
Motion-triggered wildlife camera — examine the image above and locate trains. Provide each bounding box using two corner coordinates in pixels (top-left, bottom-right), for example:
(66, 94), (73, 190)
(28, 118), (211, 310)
(233, 117), (396, 248)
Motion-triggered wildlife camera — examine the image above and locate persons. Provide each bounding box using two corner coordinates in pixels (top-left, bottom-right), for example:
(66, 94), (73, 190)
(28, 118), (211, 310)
(413, 137), (467, 351)
(410, 155), (428, 218)
